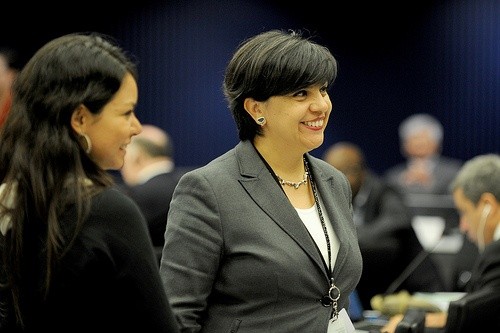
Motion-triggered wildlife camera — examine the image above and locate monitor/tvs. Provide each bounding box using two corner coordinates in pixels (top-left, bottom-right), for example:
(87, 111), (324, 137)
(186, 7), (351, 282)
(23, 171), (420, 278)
(394, 306), (425, 333)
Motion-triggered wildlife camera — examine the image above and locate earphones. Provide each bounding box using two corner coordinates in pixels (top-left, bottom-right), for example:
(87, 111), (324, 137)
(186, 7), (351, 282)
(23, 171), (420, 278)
(482, 204), (491, 217)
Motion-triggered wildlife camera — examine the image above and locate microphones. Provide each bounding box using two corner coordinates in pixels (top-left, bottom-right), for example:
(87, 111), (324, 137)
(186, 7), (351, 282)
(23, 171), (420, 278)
(387, 226), (452, 294)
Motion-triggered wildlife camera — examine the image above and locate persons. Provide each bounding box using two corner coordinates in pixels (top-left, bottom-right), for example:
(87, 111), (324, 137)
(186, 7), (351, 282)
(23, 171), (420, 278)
(160, 30), (362, 333)
(0, 32), (181, 333)
(326, 114), (500, 333)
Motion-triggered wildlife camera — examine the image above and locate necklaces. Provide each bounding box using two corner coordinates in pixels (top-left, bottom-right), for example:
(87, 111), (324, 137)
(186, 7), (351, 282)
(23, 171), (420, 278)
(277, 157), (308, 189)
